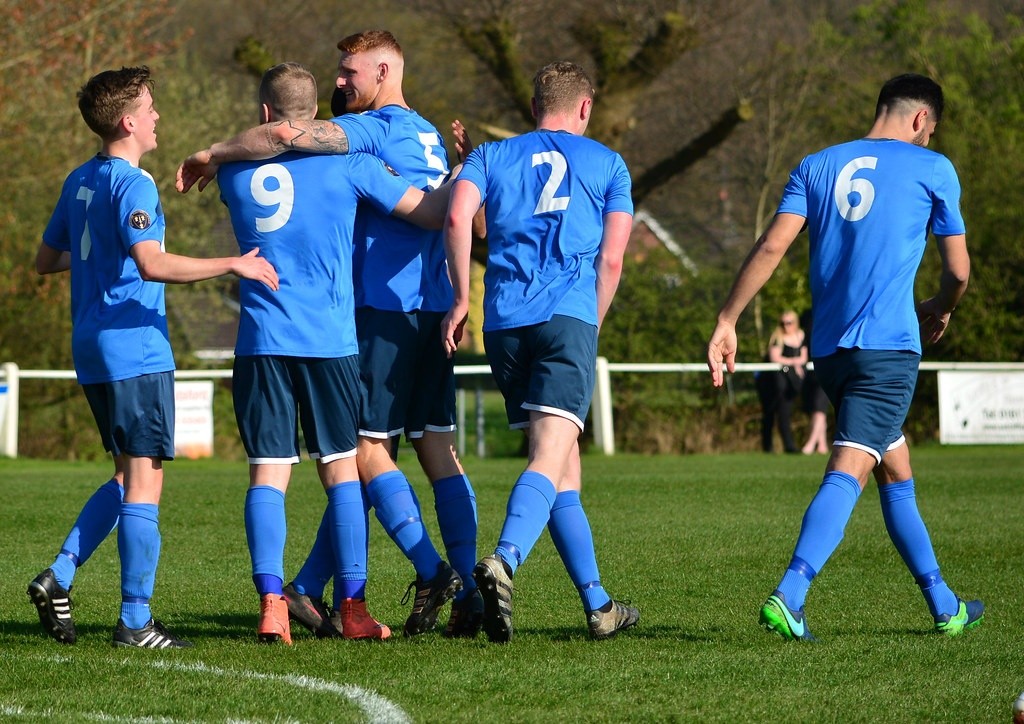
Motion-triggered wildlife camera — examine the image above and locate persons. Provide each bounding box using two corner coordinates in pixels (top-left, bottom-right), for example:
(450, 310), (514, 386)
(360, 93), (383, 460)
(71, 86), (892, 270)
(24, 63), (282, 650)
(175, 29), (484, 644)
(440, 61), (641, 644)
(706, 73), (986, 646)
(216, 60), (466, 647)
(282, 88), (473, 639)
(799, 308), (834, 456)
(755, 310), (809, 455)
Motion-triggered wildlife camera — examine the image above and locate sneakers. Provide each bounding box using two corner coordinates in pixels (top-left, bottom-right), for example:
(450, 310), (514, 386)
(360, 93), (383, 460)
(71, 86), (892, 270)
(758, 589), (816, 642)
(338, 598), (391, 640)
(587, 598), (639, 640)
(446, 585), (485, 640)
(257, 592), (292, 646)
(400, 560), (464, 637)
(471, 553), (514, 644)
(934, 598), (984, 635)
(329, 610), (344, 633)
(26, 567), (77, 644)
(282, 582), (343, 638)
(112, 616), (192, 649)
